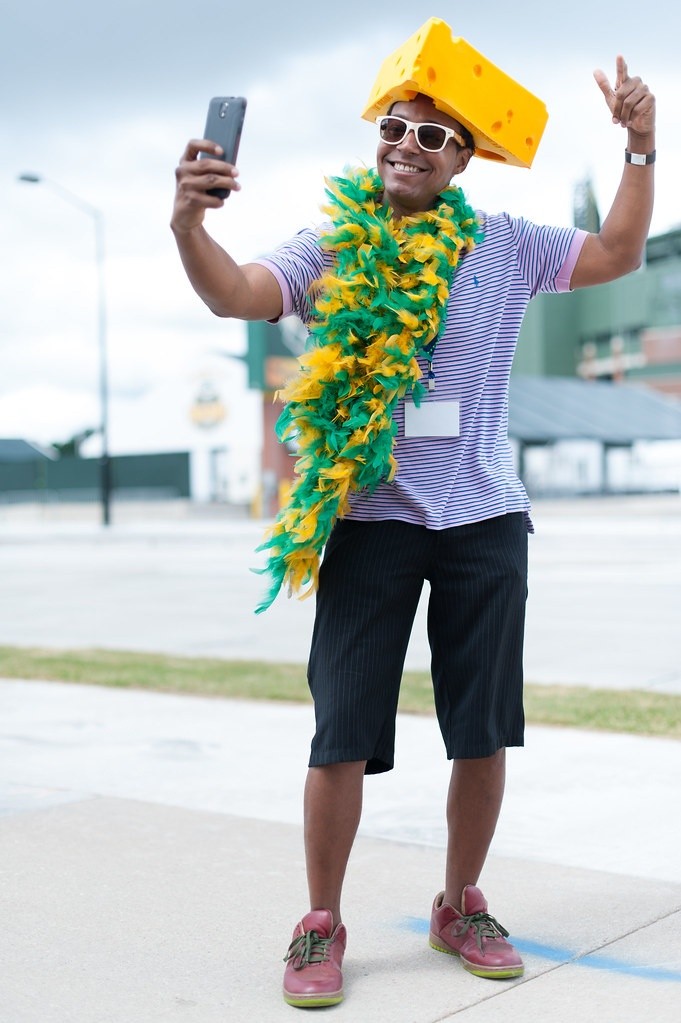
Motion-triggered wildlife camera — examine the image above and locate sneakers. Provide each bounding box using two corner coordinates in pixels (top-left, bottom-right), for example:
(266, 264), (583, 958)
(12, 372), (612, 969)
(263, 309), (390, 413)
(284, 909), (346, 1007)
(429, 884), (524, 979)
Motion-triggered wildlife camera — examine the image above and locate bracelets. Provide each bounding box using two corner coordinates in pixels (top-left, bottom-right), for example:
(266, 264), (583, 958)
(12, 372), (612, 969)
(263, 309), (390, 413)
(623, 148), (656, 166)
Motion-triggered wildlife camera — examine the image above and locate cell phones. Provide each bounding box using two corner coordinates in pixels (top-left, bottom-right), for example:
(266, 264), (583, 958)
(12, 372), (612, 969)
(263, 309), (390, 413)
(199, 95), (246, 201)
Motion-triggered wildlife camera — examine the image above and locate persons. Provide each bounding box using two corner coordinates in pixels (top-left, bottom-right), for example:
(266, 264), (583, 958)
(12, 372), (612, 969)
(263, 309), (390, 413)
(168, 18), (655, 1008)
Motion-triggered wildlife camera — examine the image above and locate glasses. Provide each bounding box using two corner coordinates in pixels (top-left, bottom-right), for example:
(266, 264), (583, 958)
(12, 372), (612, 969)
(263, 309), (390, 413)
(376, 115), (466, 154)
